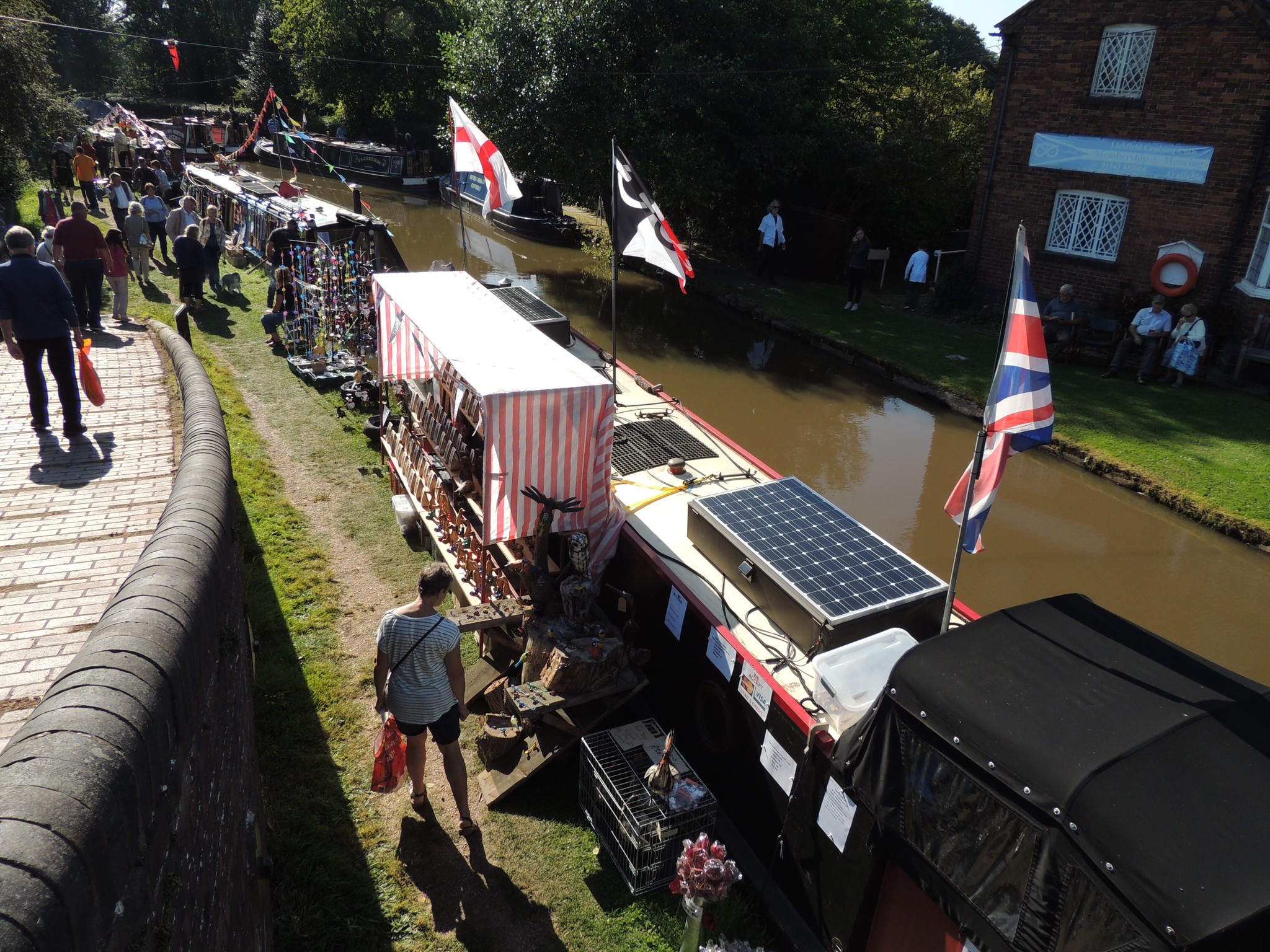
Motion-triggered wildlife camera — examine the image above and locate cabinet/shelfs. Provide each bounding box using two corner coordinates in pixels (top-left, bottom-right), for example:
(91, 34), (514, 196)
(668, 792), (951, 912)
(381, 379), (561, 648)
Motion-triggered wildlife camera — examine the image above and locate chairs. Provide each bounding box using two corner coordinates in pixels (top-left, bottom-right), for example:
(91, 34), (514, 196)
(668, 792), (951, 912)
(1040, 314), (1214, 384)
(1234, 314), (1270, 378)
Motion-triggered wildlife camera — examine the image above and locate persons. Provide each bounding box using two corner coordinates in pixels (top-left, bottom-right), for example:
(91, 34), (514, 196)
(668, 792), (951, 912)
(335, 122), (420, 176)
(373, 562), (479, 835)
(1041, 285), (1205, 391)
(842, 226), (871, 310)
(0, 105), (302, 435)
(758, 200), (787, 285)
(902, 240), (930, 312)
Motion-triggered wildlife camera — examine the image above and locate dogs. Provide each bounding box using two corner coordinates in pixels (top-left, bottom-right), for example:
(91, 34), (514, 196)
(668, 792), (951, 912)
(221, 272), (240, 295)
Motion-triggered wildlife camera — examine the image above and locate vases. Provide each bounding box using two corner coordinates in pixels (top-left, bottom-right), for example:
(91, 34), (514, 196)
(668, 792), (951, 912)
(679, 896), (715, 952)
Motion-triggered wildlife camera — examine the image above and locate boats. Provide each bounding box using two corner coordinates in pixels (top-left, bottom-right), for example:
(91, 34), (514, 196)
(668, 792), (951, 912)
(71, 97), (1270, 952)
(253, 127), (444, 191)
(439, 160), (577, 248)
(139, 102), (261, 159)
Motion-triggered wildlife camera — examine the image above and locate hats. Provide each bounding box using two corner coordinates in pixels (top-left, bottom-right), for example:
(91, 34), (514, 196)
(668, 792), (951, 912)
(76, 127), (84, 132)
(114, 127), (120, 132)
(179, 195), (197, 208)
(95, 133), (100, 136)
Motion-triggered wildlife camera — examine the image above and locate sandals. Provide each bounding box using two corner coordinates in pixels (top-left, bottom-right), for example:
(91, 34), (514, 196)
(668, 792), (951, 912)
(269, 341), (283, 347)
(409, 780), (426, 805)
(458, 816), (478, 835)
(265, 337), (274, 343)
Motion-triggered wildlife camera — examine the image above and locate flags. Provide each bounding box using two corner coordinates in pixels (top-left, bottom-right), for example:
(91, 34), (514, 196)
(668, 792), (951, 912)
(450, 95), (522, 218)
(944, 225), (1054, 555)
(612, 140), (694, 296)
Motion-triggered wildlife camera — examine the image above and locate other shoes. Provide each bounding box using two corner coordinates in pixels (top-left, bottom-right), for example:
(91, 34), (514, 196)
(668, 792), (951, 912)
(195, 307), (206, 313)
(62, 422), (87, 437)
(30, 419), (51, 431)
(112, 314), (132, 322)
(186, 305), (194, 312)
(91, 324), (105, 331)
(82, 326), (92, 333)
(144, 278), (149, 281)
(136, 276), (143, 281)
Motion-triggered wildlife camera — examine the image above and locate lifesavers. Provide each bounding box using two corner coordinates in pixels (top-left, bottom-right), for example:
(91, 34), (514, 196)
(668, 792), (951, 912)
(1151, 253), (1198, 297)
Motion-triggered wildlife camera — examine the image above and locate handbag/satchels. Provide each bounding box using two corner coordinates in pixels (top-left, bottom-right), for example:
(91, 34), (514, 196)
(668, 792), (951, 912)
(77, 338), (105, 406)
(139, 233), (154, 250)
(371, 713), (409, 795)
(383, 674), (392, 705)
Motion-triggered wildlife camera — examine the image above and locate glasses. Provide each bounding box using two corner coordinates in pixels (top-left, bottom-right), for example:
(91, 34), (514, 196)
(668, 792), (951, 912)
(96, 136), (100, 138)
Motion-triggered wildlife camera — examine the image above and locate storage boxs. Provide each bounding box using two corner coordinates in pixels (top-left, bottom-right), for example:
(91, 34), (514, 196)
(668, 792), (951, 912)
(811, 626), (919, 726)
(225, 245), (248, 268)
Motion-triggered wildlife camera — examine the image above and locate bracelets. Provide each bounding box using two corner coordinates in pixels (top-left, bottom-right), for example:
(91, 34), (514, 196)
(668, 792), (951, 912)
(759, 244), (762, 246)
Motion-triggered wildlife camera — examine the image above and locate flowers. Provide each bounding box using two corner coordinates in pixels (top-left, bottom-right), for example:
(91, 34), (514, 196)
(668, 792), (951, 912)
(670, 832), (742, 901)
